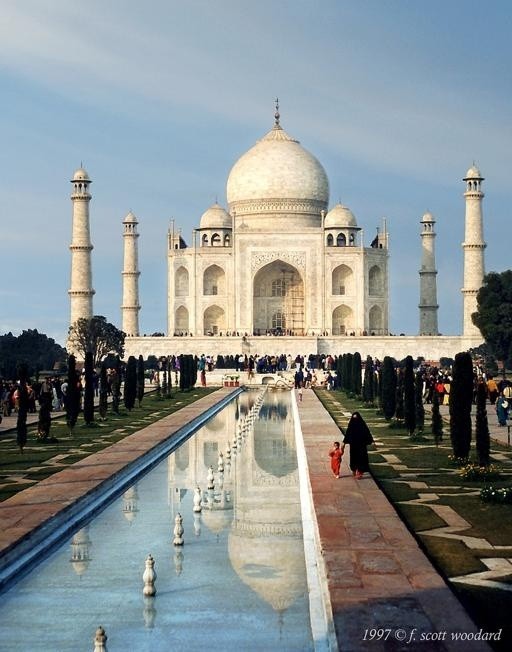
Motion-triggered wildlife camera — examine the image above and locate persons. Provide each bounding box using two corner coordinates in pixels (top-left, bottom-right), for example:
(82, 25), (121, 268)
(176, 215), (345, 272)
(343, 412), (376, 480)
(329, 442), (344, 479)
